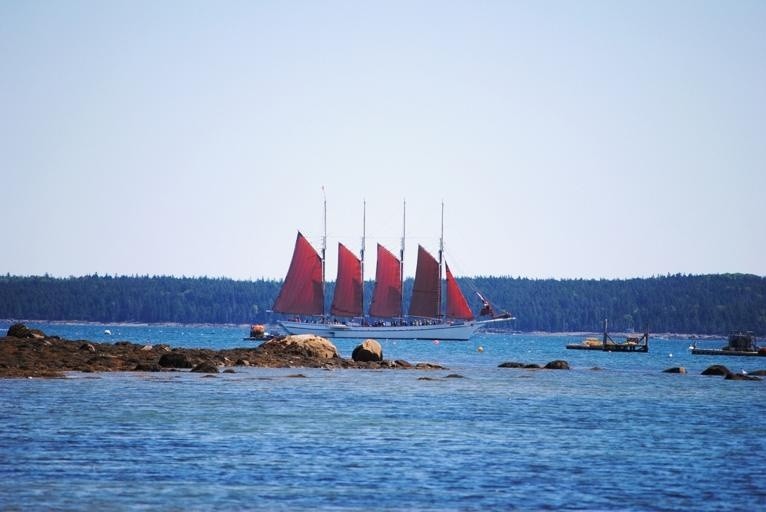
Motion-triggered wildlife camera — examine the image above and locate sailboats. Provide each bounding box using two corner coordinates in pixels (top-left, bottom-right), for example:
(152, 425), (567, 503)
(265, 186), (516, 340)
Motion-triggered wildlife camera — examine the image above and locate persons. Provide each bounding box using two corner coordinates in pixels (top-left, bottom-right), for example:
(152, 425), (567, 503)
(728, 328), (757, 352)
(288, 313), (456, 329)
(249, 321), (282, 340)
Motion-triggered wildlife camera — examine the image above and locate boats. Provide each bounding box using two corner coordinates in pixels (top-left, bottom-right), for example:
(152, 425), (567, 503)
(688, 329), (766, 356)
(243, 331), (274, 341)
(566, 319), (649, 352)
